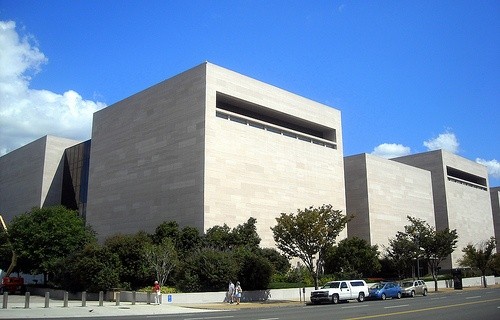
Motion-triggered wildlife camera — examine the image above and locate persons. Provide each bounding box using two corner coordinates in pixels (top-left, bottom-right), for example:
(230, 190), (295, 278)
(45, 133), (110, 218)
(229, 281), (235, 303)
(153, 281), (160, 305)
(235, 281), (243, 305)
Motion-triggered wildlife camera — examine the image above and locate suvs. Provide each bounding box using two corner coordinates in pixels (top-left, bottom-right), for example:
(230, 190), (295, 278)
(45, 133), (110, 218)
(0, 277), (25, 294)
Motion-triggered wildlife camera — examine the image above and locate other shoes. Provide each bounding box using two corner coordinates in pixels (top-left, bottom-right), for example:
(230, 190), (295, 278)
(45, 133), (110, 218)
(230, 301), (241, 304)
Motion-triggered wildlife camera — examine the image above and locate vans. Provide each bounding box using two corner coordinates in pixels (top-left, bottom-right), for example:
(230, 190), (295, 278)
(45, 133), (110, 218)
(310, 280), (369, 304)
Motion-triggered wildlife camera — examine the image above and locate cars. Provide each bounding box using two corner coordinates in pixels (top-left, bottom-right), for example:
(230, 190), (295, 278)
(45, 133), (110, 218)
(368, 281), (402, 300)
(400, 280), (427, 297)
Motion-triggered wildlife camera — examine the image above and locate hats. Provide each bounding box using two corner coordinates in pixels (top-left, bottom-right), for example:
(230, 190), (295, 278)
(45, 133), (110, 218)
(154, 280), (158, 284)
